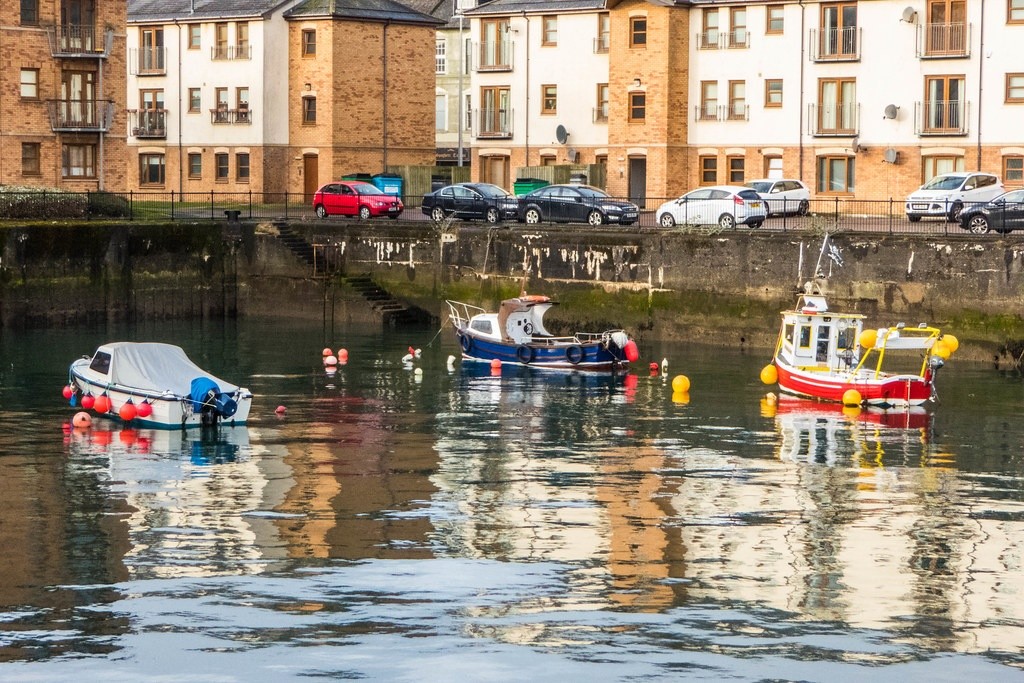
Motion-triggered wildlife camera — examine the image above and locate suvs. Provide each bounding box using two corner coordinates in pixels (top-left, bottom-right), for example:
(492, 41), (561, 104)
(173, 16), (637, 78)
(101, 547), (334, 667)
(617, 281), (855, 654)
(742, 180), (811, 217)
(904, 172), (1009, 221)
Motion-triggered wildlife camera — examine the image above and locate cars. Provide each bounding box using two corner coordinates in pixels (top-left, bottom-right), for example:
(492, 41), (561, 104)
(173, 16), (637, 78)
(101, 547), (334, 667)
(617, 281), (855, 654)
(419, 181), (517, 223)
(517, 184), (640, 227)
(655, 185), (765, 229)
(956, 190), (1024, 233)
(311, 180), (404, 221)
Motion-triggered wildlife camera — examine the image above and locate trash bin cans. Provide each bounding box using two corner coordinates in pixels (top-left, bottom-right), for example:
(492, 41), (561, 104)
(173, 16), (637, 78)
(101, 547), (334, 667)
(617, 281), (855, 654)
(513, 177), (549, 194)
(341, 174), (372, 182)
(373, 177), (404, 198)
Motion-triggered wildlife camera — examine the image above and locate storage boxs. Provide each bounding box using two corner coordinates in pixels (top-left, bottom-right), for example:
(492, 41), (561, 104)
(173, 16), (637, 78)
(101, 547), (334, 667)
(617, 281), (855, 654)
(513, 177), (550, 196)
(373, 174), (404, 199)
(342, 173), (372, 184)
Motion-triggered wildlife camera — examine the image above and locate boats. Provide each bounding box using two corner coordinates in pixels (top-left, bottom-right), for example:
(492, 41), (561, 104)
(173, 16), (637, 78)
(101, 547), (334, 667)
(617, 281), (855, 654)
(443, 292), (638, 375)
(66, 340), (254, 432)
(771, 279), (945, 410)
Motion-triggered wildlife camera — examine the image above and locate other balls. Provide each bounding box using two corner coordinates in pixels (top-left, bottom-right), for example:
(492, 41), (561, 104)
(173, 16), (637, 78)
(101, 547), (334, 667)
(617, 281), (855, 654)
(671, 374), (691, 392)
(931, 340), (951, 361)
(119, 429), (137, 447)
(93, 395), (112, 414)
(671, 391), (690, 406)
(135, 402), (153, 417)
(72, 411), (93, 428)
(81, 395), (95, 409)
(760, 364), (779, 385)
(491, 358), (502, 368)
(325, 355), (338, 366)
(62, 386), (73, 399)
(338, 348), (348, 357)
(858, 328), (878, 349)
(843, 389), (862, 408)
(843, 405), (862, 419)
(939, 332), (959, 352)
(119, 403), (136, 421)
(322, 347), (333, 356)
